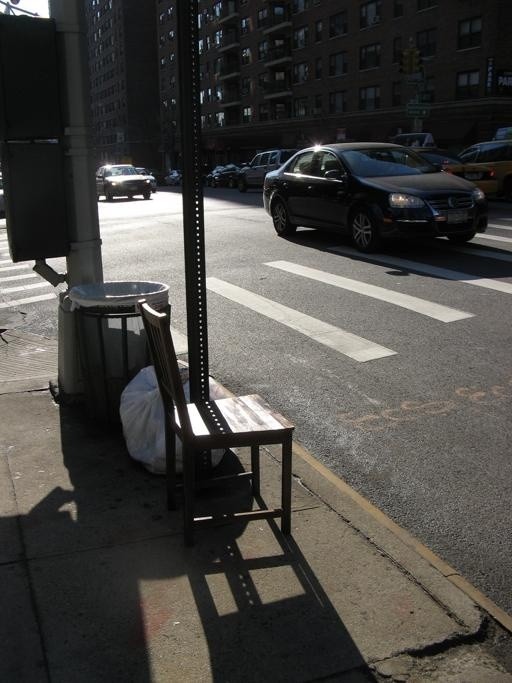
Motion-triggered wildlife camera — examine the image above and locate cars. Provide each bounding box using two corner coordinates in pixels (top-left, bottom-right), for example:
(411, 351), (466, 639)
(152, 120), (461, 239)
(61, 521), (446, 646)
(96, 163), (183, 201)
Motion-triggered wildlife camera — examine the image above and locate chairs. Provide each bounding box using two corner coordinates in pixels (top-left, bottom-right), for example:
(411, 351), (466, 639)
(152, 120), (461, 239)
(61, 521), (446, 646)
(135, 299), (297, 557)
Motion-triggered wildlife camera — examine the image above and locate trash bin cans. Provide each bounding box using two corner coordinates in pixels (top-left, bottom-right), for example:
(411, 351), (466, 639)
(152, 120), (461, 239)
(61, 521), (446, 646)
(69, 281), (171, 432)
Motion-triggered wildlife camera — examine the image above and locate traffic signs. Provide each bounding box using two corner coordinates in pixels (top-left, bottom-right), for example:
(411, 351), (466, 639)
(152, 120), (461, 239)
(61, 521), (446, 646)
(406, 78), (429, 119)
